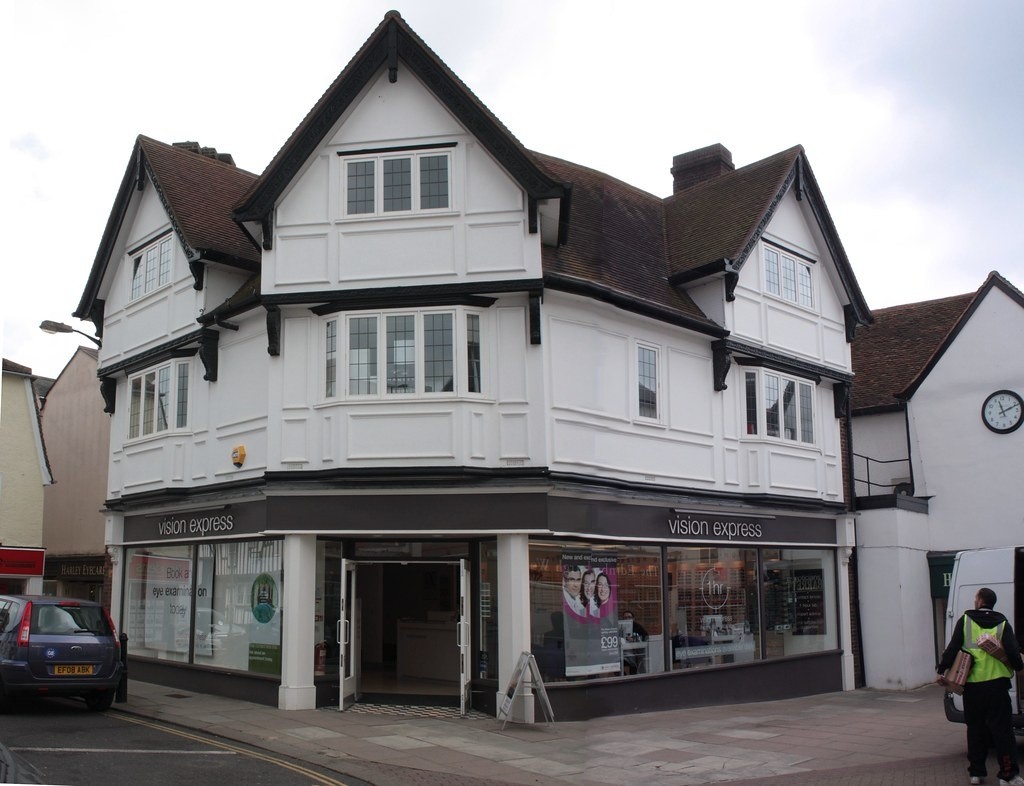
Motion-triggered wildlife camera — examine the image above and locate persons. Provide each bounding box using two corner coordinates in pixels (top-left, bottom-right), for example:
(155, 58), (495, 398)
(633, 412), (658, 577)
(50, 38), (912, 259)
(563, 564), (586, 618)
(544, 611), (577, 681)
(594, 572), (613, 617)
(614, 610), (649, 677)
(935, 588), (1024, 786)
(580, 569), (599, 617)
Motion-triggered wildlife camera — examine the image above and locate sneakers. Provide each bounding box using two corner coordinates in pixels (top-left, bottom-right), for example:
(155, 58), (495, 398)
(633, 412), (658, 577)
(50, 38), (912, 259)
(999, 776), (1024, 785)
(970, 776), (981, 784)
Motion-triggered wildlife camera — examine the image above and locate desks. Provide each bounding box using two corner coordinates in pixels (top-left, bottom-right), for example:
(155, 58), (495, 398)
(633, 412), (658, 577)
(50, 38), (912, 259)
(618, 639), (672, 678)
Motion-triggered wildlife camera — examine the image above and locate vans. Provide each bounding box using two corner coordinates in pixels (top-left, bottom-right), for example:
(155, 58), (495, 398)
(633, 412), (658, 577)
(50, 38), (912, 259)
(945, 543), (1024, 735)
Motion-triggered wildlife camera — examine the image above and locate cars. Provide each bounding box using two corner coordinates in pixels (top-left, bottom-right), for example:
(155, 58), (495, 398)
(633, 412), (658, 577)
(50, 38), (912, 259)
(0, 594), (122, 710)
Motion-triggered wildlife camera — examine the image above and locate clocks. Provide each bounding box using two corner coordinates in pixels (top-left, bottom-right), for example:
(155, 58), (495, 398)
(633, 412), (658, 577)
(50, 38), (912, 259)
(981, 389), (1024, 435)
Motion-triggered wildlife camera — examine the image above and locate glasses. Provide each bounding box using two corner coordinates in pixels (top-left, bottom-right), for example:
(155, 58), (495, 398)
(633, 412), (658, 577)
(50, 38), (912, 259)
(596, 582), (609, 589)
(625, 616), (633, 618)
(564, 577), (582, 582)
